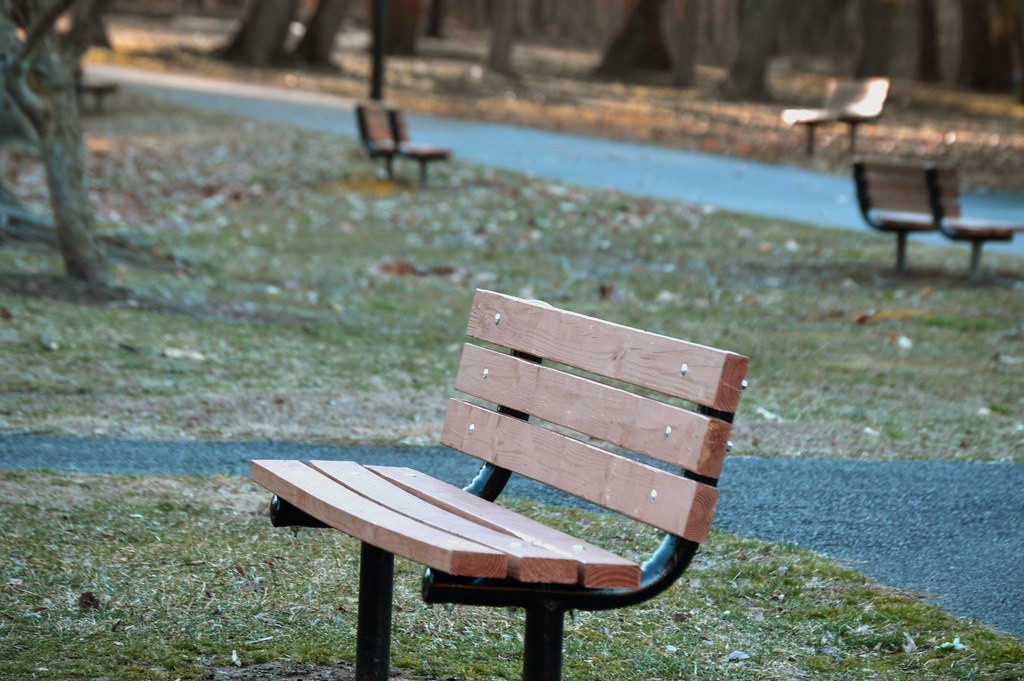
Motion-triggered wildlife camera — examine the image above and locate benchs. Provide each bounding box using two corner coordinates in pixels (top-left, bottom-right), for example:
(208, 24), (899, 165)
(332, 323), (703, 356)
(76, 78), (120, 112)
(854, 155), (1015, 273)
(782, 73), (892, 156)
(246, 287), (749, 680)
(356, 100), (449, 187)
